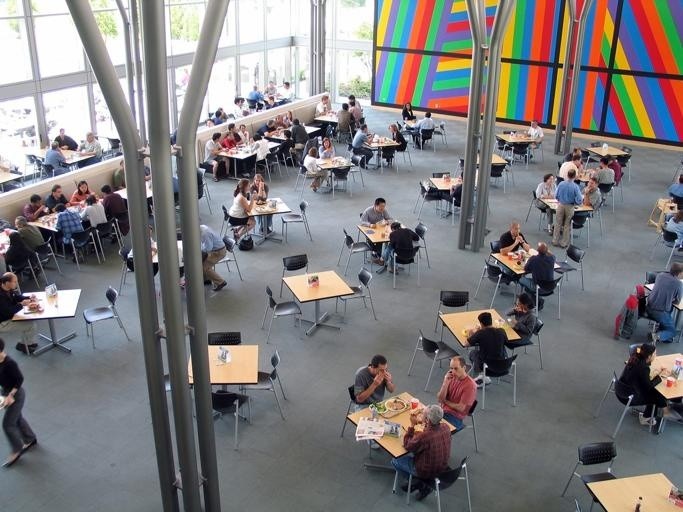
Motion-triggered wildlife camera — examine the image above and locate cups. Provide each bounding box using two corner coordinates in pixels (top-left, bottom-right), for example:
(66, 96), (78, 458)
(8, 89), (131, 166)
(675, 358), (682, 366)
(412, 399), (419, 409)
(381, 231), (385, 238)
(508, 253), (513, 260)
(667, 377), (673, 388)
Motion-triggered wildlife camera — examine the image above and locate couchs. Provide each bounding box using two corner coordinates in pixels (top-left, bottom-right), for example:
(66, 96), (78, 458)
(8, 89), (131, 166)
(75, 166), (121, 199)
(198, 122), (254, 179)
(252, 109), (296, 138)
(0, 181), (78, 228)
(295, 98), (332, 127)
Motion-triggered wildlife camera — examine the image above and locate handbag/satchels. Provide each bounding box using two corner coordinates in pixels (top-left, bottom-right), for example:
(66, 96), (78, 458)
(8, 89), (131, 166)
(239, 235), (254, 250)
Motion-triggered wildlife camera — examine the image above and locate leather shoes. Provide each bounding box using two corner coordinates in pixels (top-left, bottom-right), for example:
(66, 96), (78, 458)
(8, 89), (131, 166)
(213, 281), (227, 291)
(28, 343), (38, 348)
(16, 342), (34, 354)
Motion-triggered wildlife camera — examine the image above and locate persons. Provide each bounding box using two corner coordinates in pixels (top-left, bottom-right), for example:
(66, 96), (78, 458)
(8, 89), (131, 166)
(4, 216), (48, 280)
(506, 293), (535, 346)
(524, 120), (544, 162)
(334, 95), (362, 142)
(112, 160), (125, 191)
(437, 357), (476, 431)
(606, 154), (621, 185)
(228, 178), (256, 245)
(0, 338), (38, 468)
(304, 148), (329, 192)
(196, 168), (204, 198)
(258, 111), (293, 136)
(101, 185), (127, 246)
(143, 164), (154, 218)
(206, 96), (244, 127)
(0, 272), (38, 354)
(249, 82), (294, 114)
(545, 169), (583, 248)
(402, 103), (413, 130)
(5, 233), (28, 285)
(221, 124), (249, 149)
(171, 176), (179, 207)
(519, 242), (555, 311)
(465, 313), (509, 389)
(242, 134), (271, 177)
(615, 344), (667, 426)
(267, 130), (295, 162)
(371, 222), (419, 275)
(491, 220), (530, 256)
(443, 172), (463, 207)
(412, 112), (435, 149)
(354, 355), (396, 450)
(53, 204), (86, 263)
(78, 194), (107, 253)
(43, 184), (70, 214)
(204, 132), (234, 182)
(563, 147), (583, 169)
(352, 124), (373, 170)
(664, 174), (683, 252)
(391, 404), (451, 501)
(45, 129), (102, 174)
(559, 155), (582, 181)
(646, 262), (683, 346)
(291, 118), (309, 149)
(596, 158), (615, 184)
(250, 176), (272, 234)
(572, 177), (602, 228)
(389, 124), (407, 152)
(319, 138), (335, 186)
(535, 174), (556, 235)
(314, 94), (335, 137)
(70, 182), (97, 208)
(24, 194), (48, 221)
(358, 197), (394, 259)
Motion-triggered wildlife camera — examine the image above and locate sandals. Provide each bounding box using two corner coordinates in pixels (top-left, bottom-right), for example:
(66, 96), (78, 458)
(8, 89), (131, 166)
(22, 439), (37, 450)
(2, 449), (22, 467)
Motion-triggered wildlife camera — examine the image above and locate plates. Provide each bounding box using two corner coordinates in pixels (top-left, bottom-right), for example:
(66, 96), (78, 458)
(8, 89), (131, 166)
(369, 403), (388, 413)
(385, 399), (407, 411)
(28, 302), (38, 308)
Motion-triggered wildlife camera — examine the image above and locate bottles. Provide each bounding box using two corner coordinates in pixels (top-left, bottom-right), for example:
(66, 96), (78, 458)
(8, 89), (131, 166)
(634, 497), (642, 512)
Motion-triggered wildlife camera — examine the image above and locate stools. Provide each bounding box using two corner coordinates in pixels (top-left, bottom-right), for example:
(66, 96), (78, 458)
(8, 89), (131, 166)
(647, 198), (678, 233)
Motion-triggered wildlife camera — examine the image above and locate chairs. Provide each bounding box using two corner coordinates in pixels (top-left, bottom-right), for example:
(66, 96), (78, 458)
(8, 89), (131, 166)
(24, 154), (37, 183)
(106, 137), (120, 158)
(495, 157), (515, 185)
(620, 146), (632, 183)
(408, 222), (432, 273)
(452, 399), (478, 452)
(388, 245), (421, 289)
(219, 205), (249, 238)
(260, 285), (303, 343)
(561, 441), (618, 512)
(338, 121), (356, 143)
(487, 240), (500, 262)
(414, 181), (443, 220)
(238, 349), (287, 421)
(337, 227), (373, 276)
(525, 190), (546, 232)
(335, 266), (378, 323)
(357, 212), (370, 259)
(86, 217), (122, 262)
(492, 135), (515, 166)
(571, 212), (590, 248)
(595, 370), (656, 438)
(329, 166), (353, 198)
(581, 149), (595, 170)
(117, 246), (135, 296)
(503, 130), (517, 134)
(281, 199), (312, 242)
(629, 343), (644, 357)
(645, 271), (657, 284)
(432, 171), (451, 178)
(340, 384), (372, 458)
(211, 390), (253, 450)
(42, 163), (55, 177)
(294, 161), (322, 198)
(280, 254), (309, 302)
(406, 455), (472, 512)
(475, 258), (517, 309)
(83, 285), (130, 349)
(198, 167), (212, 220)
(554, 243), (586, 294)
(506, 318), (544, 369)
(591, 141), (601, 147)
(668, 192), (683, 210)
(246, 98), (258, 114)
(598, 182), (616, 213)
(276, 147), (296, 176)
(650, 224), (683, 270)
(21, 236), (62, 286)
(62, 226), (101, 269)
(114, 210), (130, 248)
(355, 116), (367, 132)
(213, 234), (243, 279)
(491, 163), (507, 192)
(635, 283), (659, 333)
(396, 121), (415, 148)
(453, 158), (465, 179)
(434, 121), (448, 145)
(535, 275), (564, 320)
(349, 154), (365, 188)
(419, 128), (435, 152)
(8, 250), (41, 289)
(614, 171), (625, 201)
(478, 353), (518, 409)
(207, 331), (242, 346)
(393, 137), (412, 173)
(435, 291), (469, 342)
(445, 195), (461, 224)
(31, 157), (43, 182)
(408, 328), (460, 392)
(555, 175), (565, 187)
(254, 150), (282, 183)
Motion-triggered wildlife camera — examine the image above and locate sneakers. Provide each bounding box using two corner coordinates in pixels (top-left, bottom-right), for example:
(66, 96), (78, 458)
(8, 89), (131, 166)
(399, 481), (418, 493)
(416, 487), (433, 500)
(373, 257), (384, 266)
(639, 412), (644, 420)
(485, 376), (491, 385)
(640, 417), (656, 426)
(368, 440), (380, 449)
(648, 331), (657, 344)
(389, 269), (399, 275)
(538, 298), (544, 312)
